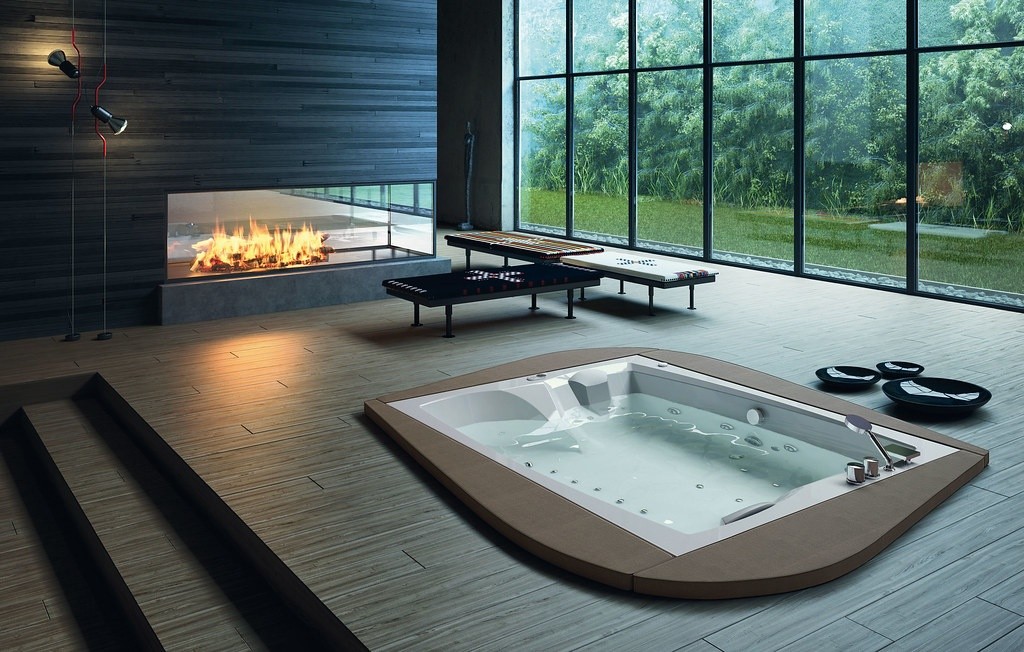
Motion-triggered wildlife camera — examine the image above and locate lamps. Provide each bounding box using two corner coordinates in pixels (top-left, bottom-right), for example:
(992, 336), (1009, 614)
(89, 104), (127, 135)
(47, 49), (80, 79)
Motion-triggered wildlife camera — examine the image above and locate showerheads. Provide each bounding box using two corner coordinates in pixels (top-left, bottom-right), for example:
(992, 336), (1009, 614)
(843, 413), (896, 472)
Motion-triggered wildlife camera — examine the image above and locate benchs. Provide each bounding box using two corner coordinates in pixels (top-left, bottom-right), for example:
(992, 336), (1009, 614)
(559, 251), (720, 318)
(382, 262), (601, 338)
(444, 232), (604, 268)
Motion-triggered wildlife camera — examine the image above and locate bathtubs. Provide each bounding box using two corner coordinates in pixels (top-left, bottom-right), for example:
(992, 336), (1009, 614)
(385, 353), (961, 556)
